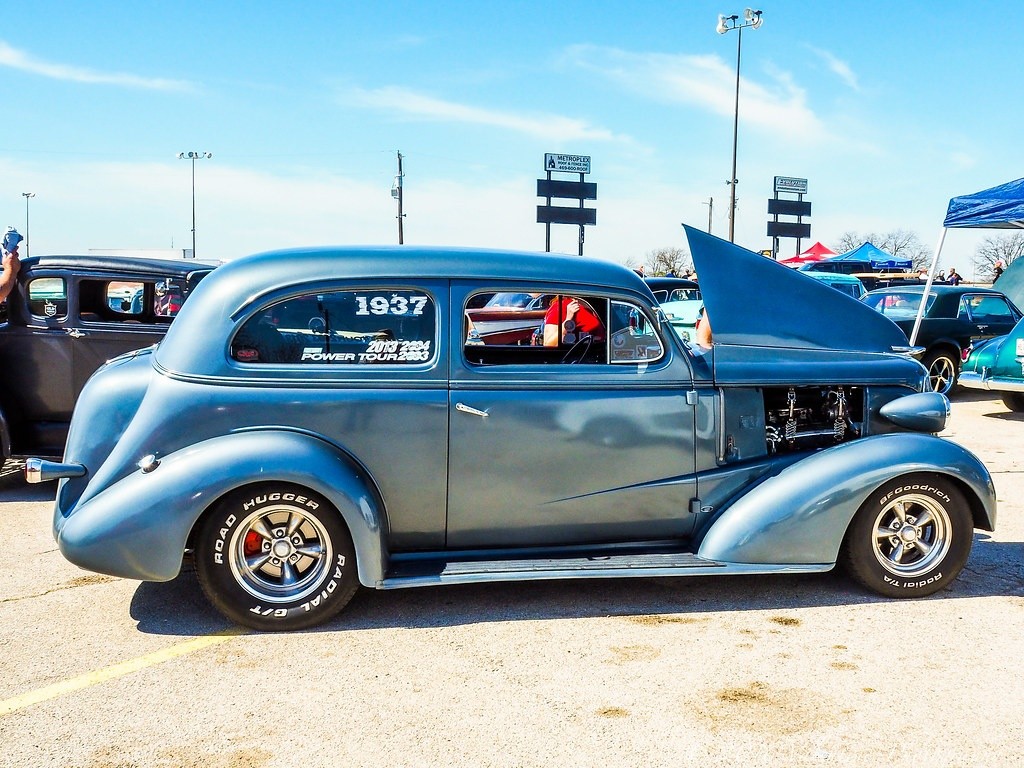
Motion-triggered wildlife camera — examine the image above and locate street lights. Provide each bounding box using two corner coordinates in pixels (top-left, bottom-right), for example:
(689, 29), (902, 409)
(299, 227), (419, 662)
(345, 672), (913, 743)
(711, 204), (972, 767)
(175, 151), (213, 258)
(714, 6), (764, 245)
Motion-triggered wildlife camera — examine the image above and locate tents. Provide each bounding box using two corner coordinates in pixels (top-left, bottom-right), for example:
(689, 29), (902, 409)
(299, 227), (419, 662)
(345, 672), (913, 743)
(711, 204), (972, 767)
(779, 241), (838, 268)
(831, 242), (913, 273)
(908, 178), (1024, 347)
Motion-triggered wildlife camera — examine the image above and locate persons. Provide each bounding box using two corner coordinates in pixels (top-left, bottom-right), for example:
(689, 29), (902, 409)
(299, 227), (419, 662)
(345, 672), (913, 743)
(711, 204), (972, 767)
(0, 246), (21, 303)
(936, 269), (945, 281)
(666, 269), (676, 278)
(947, 266), (965, 286)
(992, 260), (1003, 284)
(542, 295), (606, 347)
(640, 266), (643, 273)
(682, 269), (695, 279)
(916, 267), (929, 279)
(695, 303), (713, 349)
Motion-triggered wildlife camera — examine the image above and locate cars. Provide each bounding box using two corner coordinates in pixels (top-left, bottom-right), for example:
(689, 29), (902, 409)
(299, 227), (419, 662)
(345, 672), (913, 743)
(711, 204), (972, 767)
(271, 277), (707, 361)
(857, 254), (1024, 399)
(956, 315), (1024, 413)
(0, 254), (218, 471)
(797, 269), (868, 302)
(27, 224), (997, 636)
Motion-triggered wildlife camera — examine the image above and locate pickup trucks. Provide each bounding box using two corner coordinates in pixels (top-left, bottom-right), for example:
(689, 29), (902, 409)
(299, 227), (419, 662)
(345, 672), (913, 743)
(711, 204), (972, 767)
(794, 259), (955, 292)
